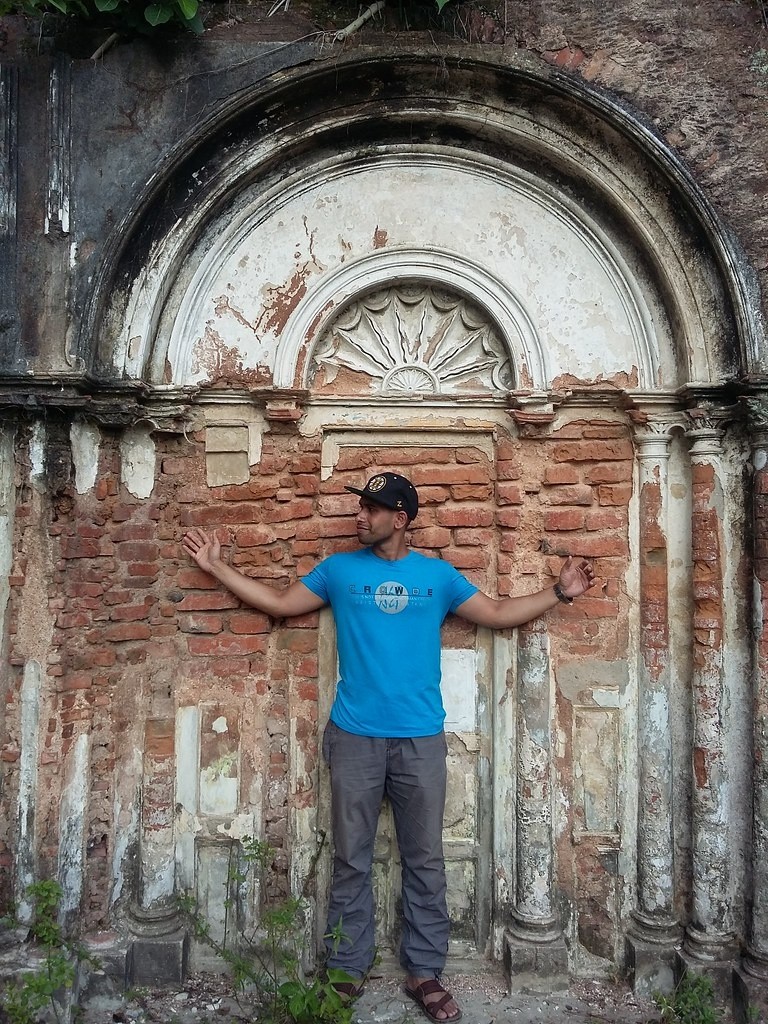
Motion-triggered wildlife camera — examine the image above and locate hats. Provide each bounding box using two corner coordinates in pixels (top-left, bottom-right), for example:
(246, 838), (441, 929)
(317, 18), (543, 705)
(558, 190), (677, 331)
(344, 472), (419, 521)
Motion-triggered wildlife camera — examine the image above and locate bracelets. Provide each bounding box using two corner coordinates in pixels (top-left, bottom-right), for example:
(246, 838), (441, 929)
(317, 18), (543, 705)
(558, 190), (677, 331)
(552, 582), (573, 604)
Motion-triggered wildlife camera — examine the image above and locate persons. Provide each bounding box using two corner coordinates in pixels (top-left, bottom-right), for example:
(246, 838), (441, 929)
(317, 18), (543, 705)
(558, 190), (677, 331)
(183, 472), (597, 1024)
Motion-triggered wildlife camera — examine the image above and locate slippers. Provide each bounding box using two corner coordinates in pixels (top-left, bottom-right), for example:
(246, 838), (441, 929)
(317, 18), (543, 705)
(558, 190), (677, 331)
(318, 982), (364, 1018)
(404, 979), (463, 1024)
